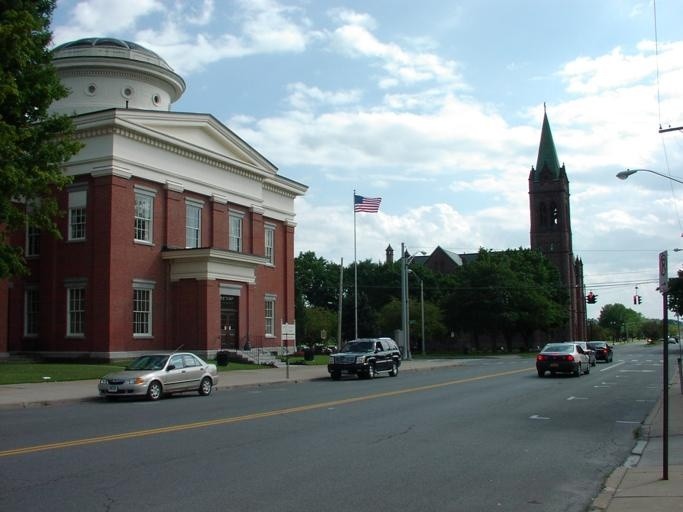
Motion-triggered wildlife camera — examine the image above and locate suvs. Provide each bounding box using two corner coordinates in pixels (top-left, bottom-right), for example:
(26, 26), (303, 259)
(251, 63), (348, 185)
(326, 337), (402, 380)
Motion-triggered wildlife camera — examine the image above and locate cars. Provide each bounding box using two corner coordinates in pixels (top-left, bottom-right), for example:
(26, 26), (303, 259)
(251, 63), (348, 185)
(537, 339), (613, 377)
(97, 353), (218, 401)
(668, 336), (679, 345)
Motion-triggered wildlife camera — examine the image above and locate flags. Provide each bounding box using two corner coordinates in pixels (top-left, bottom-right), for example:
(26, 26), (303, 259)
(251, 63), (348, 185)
(354, 194), (382, 214)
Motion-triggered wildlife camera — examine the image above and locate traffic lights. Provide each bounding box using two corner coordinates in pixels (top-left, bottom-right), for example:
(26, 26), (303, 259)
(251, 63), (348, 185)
(586, 291), (597, 304)
(633, 295), (642, 304)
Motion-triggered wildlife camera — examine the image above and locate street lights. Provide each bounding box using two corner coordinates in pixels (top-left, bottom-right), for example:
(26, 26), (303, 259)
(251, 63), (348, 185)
(615, 169), (682, 189)
(400, 243), (426, 360)
